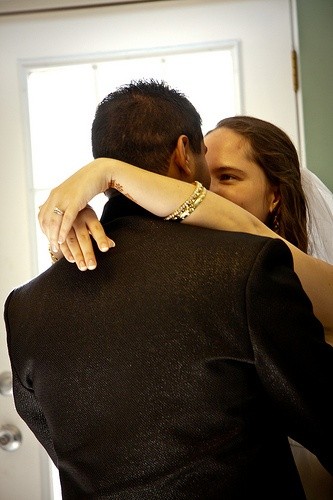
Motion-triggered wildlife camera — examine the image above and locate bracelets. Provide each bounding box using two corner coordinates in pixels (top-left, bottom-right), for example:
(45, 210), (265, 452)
(48, 243), (59, 263)
(163, 181), (206, 224)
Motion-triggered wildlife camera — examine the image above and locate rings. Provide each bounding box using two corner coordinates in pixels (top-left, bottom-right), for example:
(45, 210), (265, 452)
(53, 208), (63, 215)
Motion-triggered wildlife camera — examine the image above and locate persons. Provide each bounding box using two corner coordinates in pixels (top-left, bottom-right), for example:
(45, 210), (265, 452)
(36, 117), (333, 499)
(3, 78), (332, 499)
